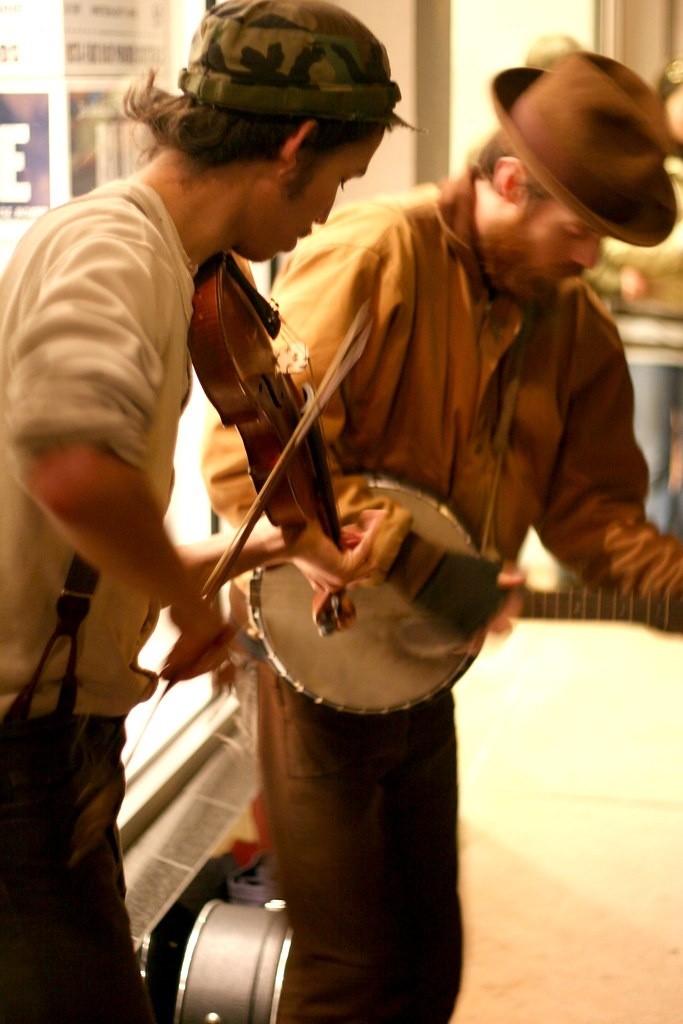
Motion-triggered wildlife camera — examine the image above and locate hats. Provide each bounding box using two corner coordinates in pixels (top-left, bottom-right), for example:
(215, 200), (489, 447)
(489, 51), (683, 247)
(178, 0), (429, 135)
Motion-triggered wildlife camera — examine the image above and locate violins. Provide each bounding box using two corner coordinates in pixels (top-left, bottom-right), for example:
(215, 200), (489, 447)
(188, 244), (356, 638)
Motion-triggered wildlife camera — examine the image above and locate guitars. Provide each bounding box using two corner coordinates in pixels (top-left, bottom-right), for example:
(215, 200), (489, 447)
(245, 476), (683, 718)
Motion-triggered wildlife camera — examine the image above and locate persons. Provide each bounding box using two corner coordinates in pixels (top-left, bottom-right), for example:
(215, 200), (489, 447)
(0, 2), (382, 1024)
(203, 35), (683, 1024)
